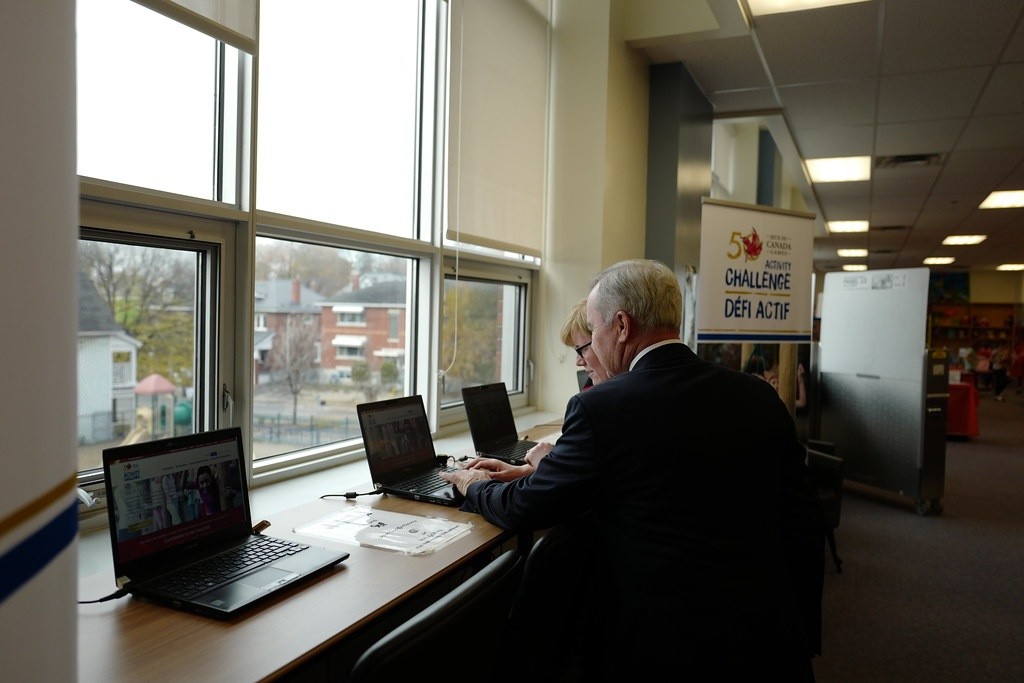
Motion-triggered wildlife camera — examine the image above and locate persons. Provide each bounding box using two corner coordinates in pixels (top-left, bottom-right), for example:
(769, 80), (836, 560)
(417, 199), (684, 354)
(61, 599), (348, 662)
(439, 260), (812, 683)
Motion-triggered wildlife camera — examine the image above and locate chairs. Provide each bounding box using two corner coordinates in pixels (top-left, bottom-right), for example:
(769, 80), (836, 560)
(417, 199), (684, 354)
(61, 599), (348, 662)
(806, 438), (847, 574)
(346, 509), (602, 683)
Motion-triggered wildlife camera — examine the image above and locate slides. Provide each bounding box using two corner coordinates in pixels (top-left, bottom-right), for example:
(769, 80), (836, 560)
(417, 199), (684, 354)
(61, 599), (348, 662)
(121, 427), (146, 446)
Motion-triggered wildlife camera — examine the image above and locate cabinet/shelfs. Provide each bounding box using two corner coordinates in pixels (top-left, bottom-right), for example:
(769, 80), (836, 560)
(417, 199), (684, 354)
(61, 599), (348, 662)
(926, 303), (1024, 352)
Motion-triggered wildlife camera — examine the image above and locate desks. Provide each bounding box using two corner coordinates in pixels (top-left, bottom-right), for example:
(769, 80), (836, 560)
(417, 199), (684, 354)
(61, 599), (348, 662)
(947, 381), (979, 440)
(960, 372), (976, 384)
(79, 411), (564, 683)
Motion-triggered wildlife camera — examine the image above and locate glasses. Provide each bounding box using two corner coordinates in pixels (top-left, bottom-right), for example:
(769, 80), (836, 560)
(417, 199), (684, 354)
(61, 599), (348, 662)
(575, 342), (592, 358)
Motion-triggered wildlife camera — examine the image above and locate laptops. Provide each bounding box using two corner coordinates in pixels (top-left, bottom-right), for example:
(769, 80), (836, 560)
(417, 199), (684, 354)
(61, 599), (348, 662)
(356, 395), (496, 507)
(577, 370), (590, 392)
(102, 426), (350, 619)
(462, 382), (539, 466)
